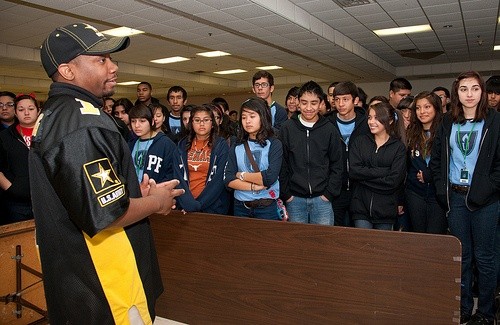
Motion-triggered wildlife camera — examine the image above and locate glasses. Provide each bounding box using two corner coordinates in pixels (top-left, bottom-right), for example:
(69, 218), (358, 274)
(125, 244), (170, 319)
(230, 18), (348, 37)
(0, 103), (14, 108)
(254, 83), (269, 88)
(215, 116), (221, 120)
(192, 118), (212, 123)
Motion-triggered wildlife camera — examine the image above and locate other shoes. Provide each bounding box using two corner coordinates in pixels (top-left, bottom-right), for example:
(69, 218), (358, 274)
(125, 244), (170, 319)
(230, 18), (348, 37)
(460, 308), (500, 325)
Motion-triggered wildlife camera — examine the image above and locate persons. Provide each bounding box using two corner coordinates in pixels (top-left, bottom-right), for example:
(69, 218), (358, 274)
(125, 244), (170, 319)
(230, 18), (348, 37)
(428, 72), (500, 325)
(28, 23), (185, 325)
(106, 70), (500, 235)
(0, 91), (42, 225)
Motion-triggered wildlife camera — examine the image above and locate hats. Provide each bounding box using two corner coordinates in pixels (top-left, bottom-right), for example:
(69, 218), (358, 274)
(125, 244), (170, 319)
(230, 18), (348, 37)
(40, 23), (130, 78)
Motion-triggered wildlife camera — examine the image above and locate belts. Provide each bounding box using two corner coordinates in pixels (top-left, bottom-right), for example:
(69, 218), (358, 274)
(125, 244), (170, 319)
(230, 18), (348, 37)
(244, 199), (273, 209)
(449, 183), (469, 192)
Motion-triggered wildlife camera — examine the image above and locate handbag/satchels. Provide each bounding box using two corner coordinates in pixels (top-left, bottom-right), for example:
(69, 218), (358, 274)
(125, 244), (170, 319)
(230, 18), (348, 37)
(277, 199), (288, 221)
